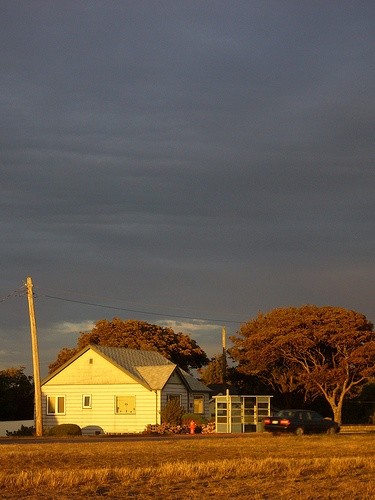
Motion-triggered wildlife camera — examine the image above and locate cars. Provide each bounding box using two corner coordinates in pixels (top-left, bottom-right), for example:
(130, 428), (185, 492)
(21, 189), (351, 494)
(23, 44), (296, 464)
(263, 409), (341, 436)
